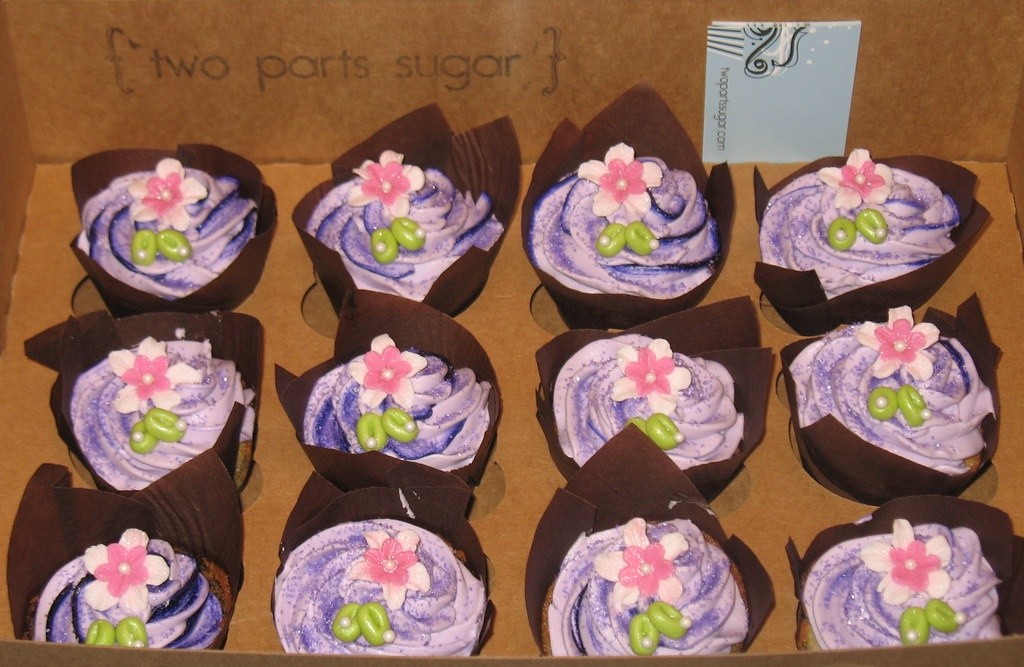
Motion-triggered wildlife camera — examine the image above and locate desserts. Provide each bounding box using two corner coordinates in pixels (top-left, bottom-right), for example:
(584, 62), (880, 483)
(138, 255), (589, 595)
(1, 141), (1023, 657)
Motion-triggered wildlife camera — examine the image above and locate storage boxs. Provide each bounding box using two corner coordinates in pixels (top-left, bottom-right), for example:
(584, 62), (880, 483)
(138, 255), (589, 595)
(0, 1), (1024, 667)
